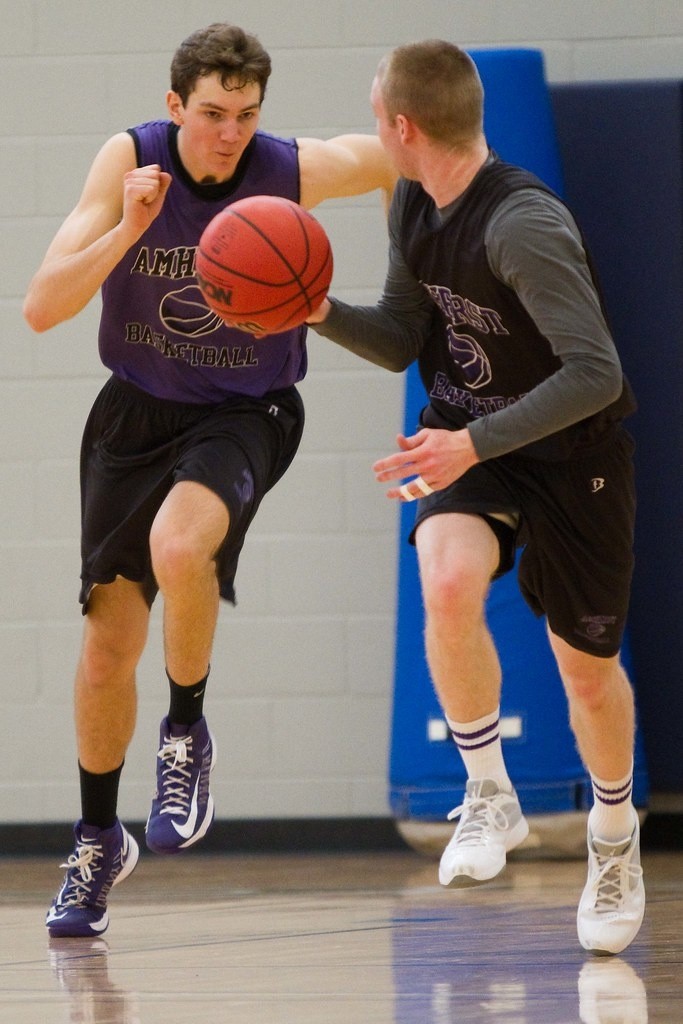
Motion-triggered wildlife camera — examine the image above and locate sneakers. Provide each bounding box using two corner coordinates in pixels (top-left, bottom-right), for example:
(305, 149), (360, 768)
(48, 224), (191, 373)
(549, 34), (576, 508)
(145, 717), (223, 855)
(438, 781), (530, 888)
(577, 797), (646, 956)
(47, 817), (140, 938)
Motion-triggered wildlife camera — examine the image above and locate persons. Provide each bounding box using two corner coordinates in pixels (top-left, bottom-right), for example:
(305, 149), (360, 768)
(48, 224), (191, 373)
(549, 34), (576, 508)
(20, 20), (402, 940)
(225, 37), (648, 959)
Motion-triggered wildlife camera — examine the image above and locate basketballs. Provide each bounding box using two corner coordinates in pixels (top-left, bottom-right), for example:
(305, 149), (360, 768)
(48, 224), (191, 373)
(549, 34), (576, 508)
(191, 194), (335, 336)
